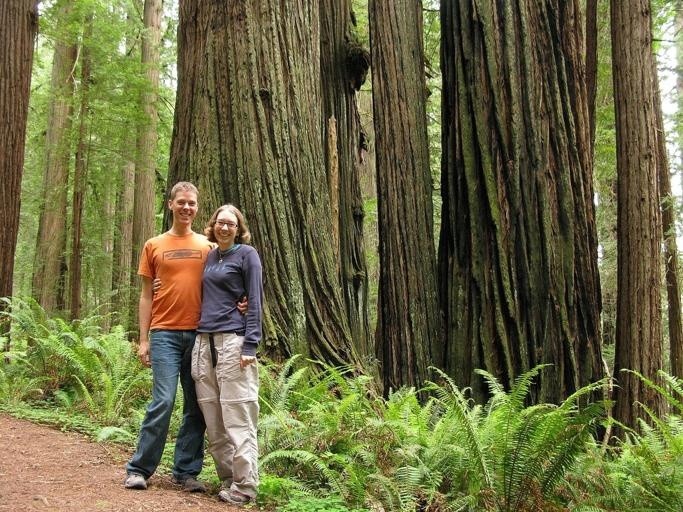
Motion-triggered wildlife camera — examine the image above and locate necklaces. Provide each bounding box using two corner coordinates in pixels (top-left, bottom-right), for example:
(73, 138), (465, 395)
(218, 249), (234, 264)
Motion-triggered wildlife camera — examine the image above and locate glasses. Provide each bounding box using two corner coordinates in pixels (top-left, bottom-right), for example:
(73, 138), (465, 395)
(215, 220), (239, 228)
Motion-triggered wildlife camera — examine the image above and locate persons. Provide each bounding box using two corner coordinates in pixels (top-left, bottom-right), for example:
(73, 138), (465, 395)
(151, 203), (264, 506)
(124, 180), (248, 494)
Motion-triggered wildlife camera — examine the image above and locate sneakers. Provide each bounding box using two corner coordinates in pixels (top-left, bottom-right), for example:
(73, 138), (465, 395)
(173, 475), (208, 493)
(124, 473), (147, 490)
(219, 479), (252, 504)
